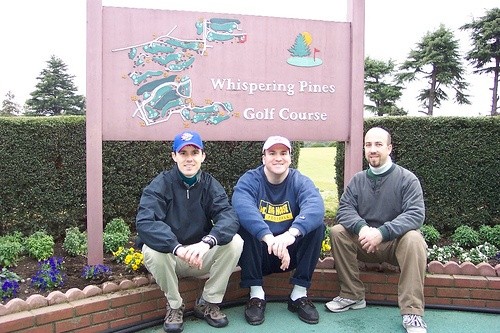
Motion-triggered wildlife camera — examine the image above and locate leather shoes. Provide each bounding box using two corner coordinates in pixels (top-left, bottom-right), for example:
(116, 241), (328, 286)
(245, 292), (267, 325)
(288, 295), (319, 324)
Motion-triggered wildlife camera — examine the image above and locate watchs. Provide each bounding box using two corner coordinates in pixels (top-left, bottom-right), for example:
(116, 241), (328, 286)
(288, 228), (299, 238)
(201, 235), (214, 249)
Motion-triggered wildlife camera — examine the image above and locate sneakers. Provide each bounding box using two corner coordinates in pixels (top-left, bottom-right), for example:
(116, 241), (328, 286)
(402, 314), (428, 333)
(164, 302), (185, 333)
(325, 296), (367, 313)
(193, 298), (229, 328)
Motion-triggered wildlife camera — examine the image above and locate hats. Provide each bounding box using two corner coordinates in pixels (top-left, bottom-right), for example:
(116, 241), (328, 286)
(263, 136), (291, 154)
(173, 131), (203, 154)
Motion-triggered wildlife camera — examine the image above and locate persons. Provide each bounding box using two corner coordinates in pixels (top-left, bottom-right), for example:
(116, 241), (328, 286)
(325, 127), (429, 333)
(136, 130), (244, 333)
(232, 135), (326, 325)
